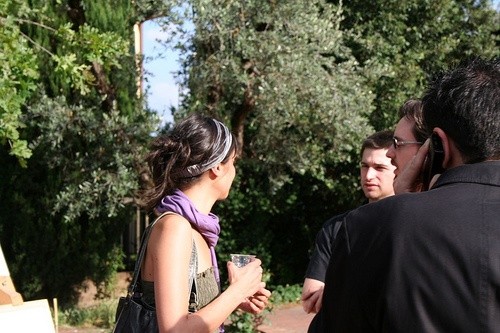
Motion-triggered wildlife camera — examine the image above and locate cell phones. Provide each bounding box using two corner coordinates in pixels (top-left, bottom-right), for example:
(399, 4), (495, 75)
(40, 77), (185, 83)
(421, 131), (444, 191)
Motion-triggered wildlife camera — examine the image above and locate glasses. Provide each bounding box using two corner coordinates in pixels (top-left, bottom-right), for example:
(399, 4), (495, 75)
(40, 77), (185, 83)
(392, 138), (423, 149)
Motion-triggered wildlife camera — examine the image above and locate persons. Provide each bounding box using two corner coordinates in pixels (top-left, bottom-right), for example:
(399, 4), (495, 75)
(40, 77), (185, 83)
(306, 65), (500, 333)
(113, 117), (273, 333)
(386, 100), (435, 196)
(302, 131), (394, 333)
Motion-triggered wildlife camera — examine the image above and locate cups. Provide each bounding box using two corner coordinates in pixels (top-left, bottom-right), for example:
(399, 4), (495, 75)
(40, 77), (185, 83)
(230, 254), (256, 268)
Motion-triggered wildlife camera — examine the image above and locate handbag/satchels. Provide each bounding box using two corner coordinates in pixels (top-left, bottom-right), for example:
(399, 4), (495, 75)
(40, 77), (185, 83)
(112, 211), (199, 333)
